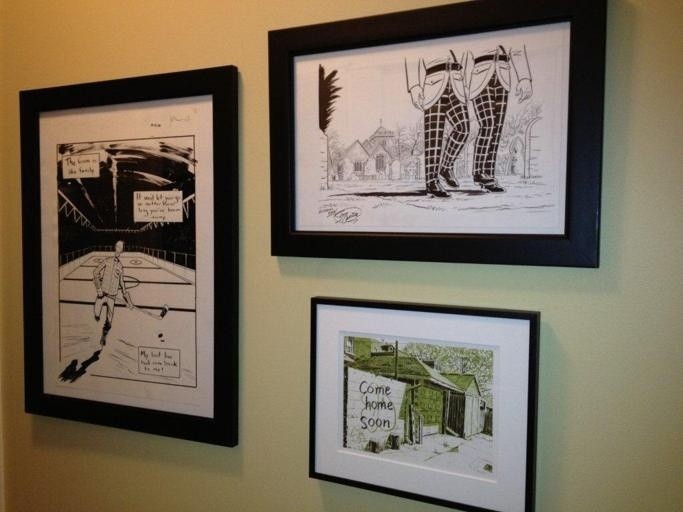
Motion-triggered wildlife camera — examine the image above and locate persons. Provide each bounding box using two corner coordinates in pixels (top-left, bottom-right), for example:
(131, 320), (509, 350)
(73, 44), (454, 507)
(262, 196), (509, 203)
(405, 43), (532, 199)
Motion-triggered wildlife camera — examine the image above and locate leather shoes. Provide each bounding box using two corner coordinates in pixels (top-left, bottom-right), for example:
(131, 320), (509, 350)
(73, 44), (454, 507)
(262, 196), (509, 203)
(427, 191), (450, 197)
(440, 167), (460, 187)
(474, 175), (496, 185)
(481, 184), (504, 192)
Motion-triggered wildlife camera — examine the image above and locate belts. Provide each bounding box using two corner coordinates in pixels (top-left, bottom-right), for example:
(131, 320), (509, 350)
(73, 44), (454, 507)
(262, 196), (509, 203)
(426, 62), (463, 76)
(475, 54), (509, 64)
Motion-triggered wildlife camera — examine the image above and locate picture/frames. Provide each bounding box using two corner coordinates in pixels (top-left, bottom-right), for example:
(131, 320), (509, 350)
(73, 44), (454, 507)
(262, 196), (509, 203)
(307, 294), (542, 511)
(19, 64), (240, 449)
(267, 0), (609, 269)
